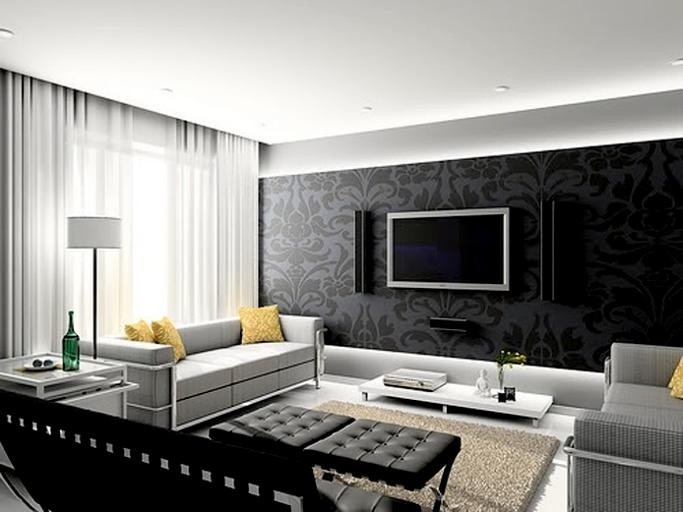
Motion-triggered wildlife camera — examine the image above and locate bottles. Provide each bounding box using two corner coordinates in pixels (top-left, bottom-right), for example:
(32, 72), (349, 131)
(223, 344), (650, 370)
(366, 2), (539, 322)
(61, 310), (80, 371)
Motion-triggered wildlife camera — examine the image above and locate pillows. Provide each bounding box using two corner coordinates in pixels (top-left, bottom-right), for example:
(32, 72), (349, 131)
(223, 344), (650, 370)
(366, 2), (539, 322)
(236, 302), (287, 347)
(123, 318), (154, 343)
(665, 354), (683, 400)
(148, 315), (188, 364)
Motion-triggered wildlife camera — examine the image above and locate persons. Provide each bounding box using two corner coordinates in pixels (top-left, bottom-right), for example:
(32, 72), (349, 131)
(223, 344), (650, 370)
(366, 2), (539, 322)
(473, 368), (491, 398)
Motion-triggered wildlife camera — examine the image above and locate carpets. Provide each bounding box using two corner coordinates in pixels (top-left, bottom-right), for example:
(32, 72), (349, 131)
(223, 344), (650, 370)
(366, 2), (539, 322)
(303, 398), (562, 512)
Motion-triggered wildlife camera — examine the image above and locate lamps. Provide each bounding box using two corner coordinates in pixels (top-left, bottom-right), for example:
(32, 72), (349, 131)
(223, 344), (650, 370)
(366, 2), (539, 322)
(65, 212), (126, 363)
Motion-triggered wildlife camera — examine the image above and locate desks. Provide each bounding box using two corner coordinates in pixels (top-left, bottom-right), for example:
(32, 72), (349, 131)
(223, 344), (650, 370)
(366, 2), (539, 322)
(357, 371), (556, 429)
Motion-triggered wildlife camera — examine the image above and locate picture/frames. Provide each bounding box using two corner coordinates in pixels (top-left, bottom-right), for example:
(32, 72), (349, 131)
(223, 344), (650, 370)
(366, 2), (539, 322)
(502, 386), (515, 402)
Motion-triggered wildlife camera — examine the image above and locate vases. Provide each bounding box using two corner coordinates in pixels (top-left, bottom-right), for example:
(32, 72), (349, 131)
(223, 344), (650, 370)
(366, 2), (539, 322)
(498, 367), (504, 390)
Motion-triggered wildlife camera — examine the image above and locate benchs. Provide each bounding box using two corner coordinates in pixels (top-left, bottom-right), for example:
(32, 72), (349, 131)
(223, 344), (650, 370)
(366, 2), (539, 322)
(204, 401), (466, 512)
(0, 387), (426, 512)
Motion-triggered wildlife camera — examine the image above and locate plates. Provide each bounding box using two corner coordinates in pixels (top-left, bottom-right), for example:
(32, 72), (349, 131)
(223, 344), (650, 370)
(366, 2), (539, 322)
(22, 360), (58, 371)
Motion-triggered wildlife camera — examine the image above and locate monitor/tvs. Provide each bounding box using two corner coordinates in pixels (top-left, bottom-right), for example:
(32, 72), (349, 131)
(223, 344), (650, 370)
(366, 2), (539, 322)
(386, 208), (510, 292)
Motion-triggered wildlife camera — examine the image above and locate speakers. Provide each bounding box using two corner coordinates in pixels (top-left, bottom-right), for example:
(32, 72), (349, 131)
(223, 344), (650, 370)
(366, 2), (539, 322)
(541, 199), (555, 301)
(354, 210), (373, 294)
(430, 317), (467, 332)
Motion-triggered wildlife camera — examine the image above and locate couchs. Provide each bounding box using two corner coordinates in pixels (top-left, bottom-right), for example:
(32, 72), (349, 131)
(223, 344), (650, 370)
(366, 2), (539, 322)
(77, 303), (329, 433)
(566, 336), (683, 511)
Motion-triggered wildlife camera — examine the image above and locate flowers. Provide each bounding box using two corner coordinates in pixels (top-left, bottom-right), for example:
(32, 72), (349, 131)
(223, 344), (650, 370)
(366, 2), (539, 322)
(497, 347), (526, 389)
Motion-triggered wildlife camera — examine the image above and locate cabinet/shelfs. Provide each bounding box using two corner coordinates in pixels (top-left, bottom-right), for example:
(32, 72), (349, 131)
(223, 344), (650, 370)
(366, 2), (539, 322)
(0, 350), (141, 477)
